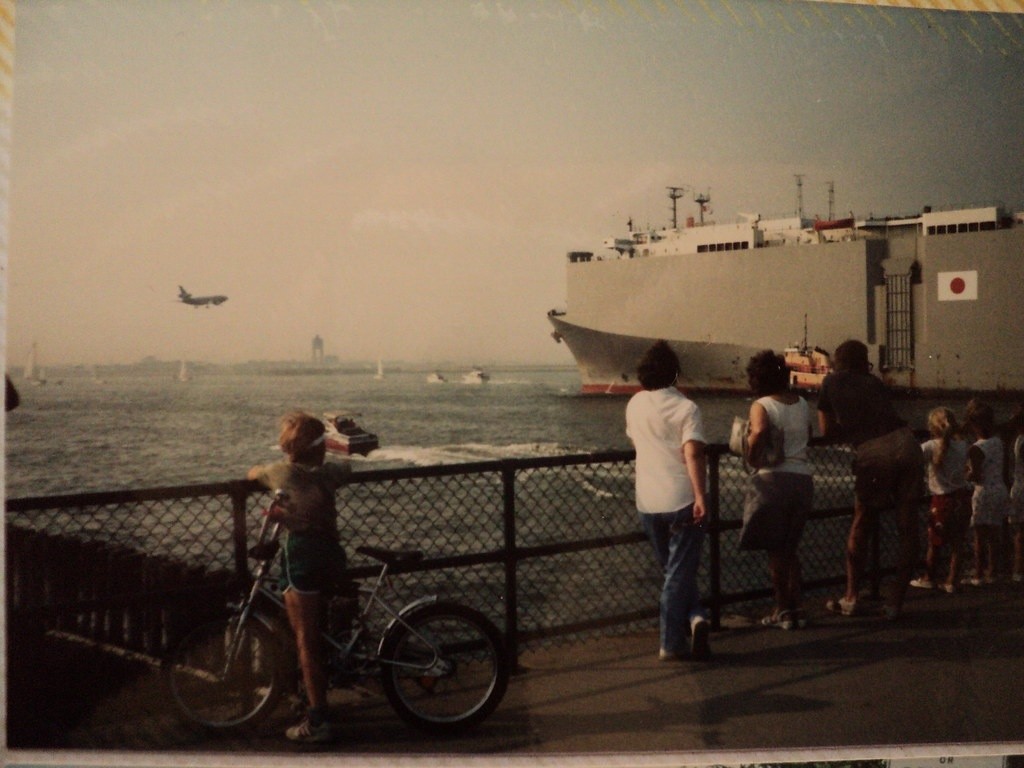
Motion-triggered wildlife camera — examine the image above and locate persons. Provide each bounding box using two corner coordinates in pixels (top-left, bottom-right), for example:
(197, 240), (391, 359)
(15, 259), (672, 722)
(735, 348), (815, 629)
(246, 412), (349, 743)
(909, 399), (1024, 594)
(817, 337), (924, 620)
(624, 339), (711, 662)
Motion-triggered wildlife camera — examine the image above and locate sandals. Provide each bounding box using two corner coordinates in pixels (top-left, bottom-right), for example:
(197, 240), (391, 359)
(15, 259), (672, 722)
(762, 610), (793, 631)
(794, 608), (809, 627)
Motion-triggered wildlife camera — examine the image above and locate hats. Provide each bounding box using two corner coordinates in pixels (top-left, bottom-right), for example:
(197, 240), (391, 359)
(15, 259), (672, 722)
(728, 416), (761, 476)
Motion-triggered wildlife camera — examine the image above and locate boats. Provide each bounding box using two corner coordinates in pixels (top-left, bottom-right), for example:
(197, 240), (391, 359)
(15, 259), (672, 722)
(780, 313), (832, 393)
(319, 411), (381, 457)
(462, 368), (490, 384)
(428, 372), (450, 383)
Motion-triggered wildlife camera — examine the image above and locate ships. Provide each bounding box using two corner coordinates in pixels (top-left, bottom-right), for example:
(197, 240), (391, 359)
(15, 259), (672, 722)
(542, 173), (1024, 401)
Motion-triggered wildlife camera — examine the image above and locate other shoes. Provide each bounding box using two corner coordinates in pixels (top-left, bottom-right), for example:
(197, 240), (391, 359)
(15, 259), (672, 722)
(1012, 572), (1024, 582)
(910, 578), (935, 589)
(691, 616), (710, 659)
(826, 597), (859, 616)
(658, 647), (689, 661)
(960, 575), (983, 586)
(936, 580), (956, 594)
(984, 576), (996, 584)
(881, 598), (899, 622)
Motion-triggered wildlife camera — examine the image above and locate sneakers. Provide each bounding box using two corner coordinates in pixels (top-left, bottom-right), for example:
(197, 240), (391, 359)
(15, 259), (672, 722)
(285, 719), (330, 742)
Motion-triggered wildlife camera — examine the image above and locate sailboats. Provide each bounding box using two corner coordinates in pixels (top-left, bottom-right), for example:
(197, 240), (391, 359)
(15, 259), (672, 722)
(373, 358), (386, 382)
(171, 356), (191, 383)
(23, 344), (47, 386)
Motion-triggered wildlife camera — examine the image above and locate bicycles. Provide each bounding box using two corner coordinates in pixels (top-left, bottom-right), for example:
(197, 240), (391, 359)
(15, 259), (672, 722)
(164, 489), (512, 735)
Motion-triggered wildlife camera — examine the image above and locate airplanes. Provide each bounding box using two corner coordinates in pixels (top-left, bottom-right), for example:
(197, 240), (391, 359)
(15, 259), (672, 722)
(177, 285), (229, 307)
